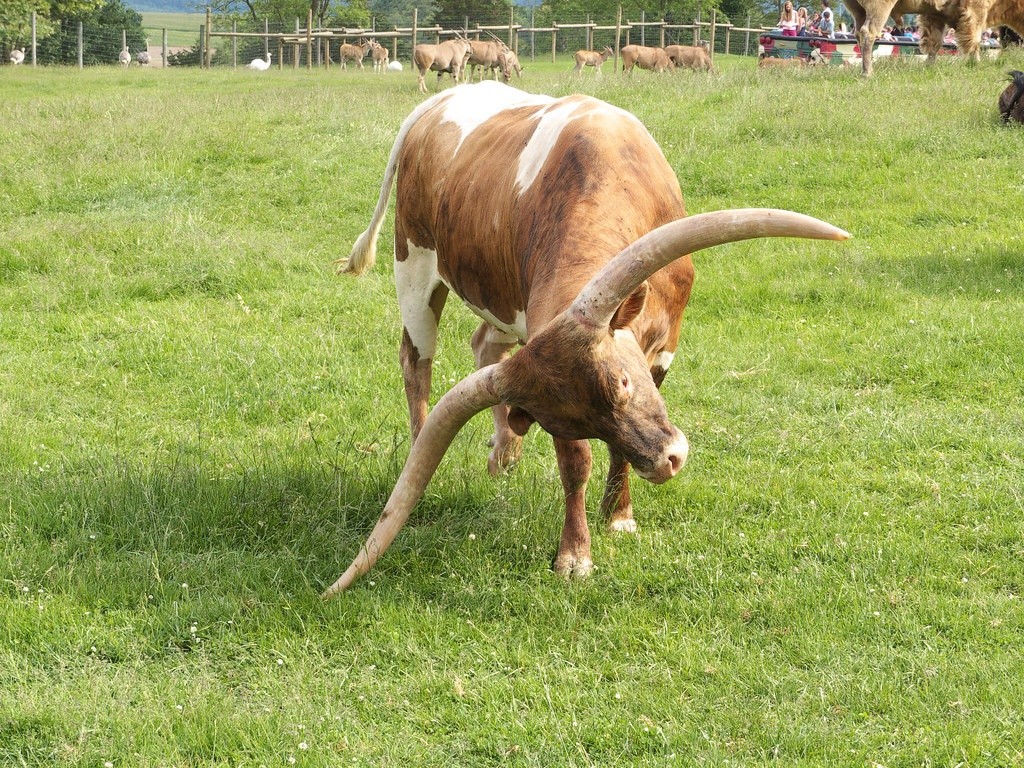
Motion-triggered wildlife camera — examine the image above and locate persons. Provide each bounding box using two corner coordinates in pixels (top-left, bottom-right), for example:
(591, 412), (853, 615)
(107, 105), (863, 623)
(776, 0), (854, 39)
(880, 23), (999, 48)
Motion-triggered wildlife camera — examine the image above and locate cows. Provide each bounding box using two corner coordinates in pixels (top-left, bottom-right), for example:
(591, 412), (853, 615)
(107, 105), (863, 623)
(842, 0), (1024, 79)
(319, 80), (853, 598)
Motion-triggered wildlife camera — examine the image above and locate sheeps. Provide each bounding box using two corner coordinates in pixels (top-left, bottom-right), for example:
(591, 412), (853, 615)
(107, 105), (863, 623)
(413, 26), (524, 94)
(759, 58), (805, 70)
(664, 44), (712, 74)
(571, 46), (614, 75)
(371, 40), (389, 74)
(339, 38), (374, 71)
(621, 46), (676, 76)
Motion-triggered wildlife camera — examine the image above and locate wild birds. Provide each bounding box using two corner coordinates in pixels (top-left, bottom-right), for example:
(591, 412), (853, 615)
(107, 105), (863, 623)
(389, 61), (402, 71)
(250, 53), (272, 71)
(10, 47), (25, 64)
(135, 42), (151, 66)
(119, 46), (132, 68)
